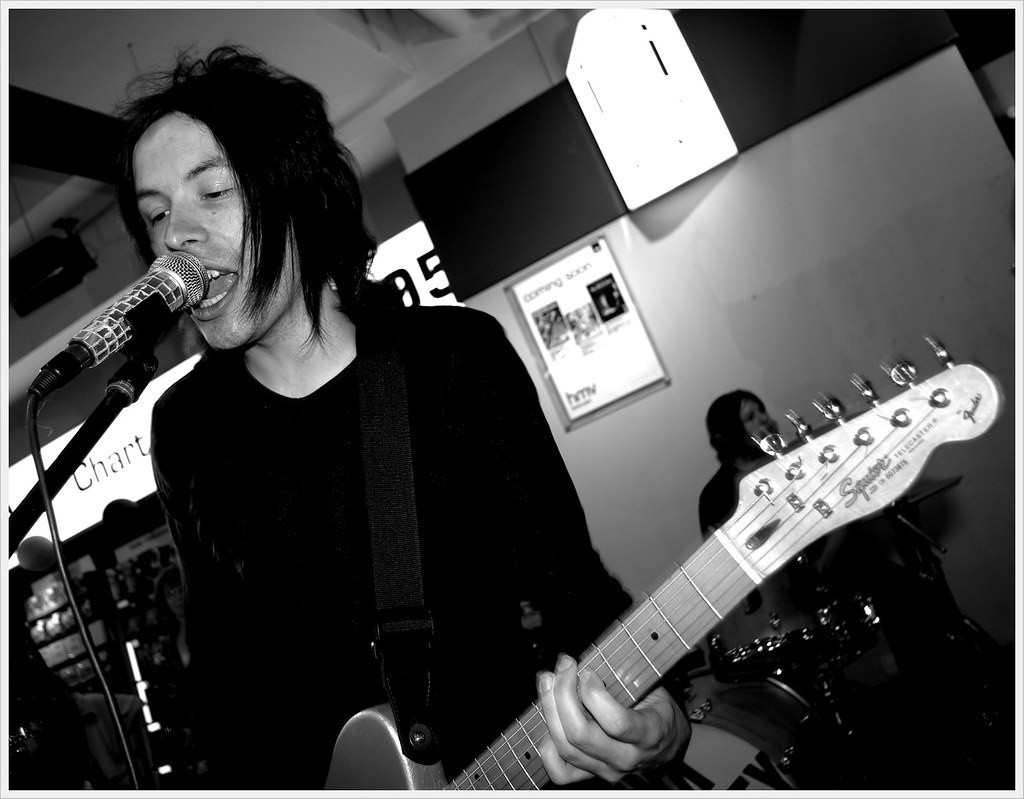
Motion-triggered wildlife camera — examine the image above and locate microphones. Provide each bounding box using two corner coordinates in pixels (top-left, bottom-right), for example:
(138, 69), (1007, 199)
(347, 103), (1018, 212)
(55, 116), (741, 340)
(28, 252), (209, 402)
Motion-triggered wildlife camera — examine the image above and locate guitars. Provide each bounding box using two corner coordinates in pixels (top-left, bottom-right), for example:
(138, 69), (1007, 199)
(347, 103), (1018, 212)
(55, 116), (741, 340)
(319, 329), (1003, 791)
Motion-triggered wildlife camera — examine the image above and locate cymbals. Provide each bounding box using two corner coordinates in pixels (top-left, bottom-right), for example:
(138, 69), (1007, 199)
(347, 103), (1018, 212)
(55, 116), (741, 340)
(899, 470), (963, 509)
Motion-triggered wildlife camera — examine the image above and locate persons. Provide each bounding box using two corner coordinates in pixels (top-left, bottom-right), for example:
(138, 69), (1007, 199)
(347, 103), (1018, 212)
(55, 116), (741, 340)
(154, 566), (196, 683)
(699, 390), (875, 575)
(9, 38), (692, 792)
